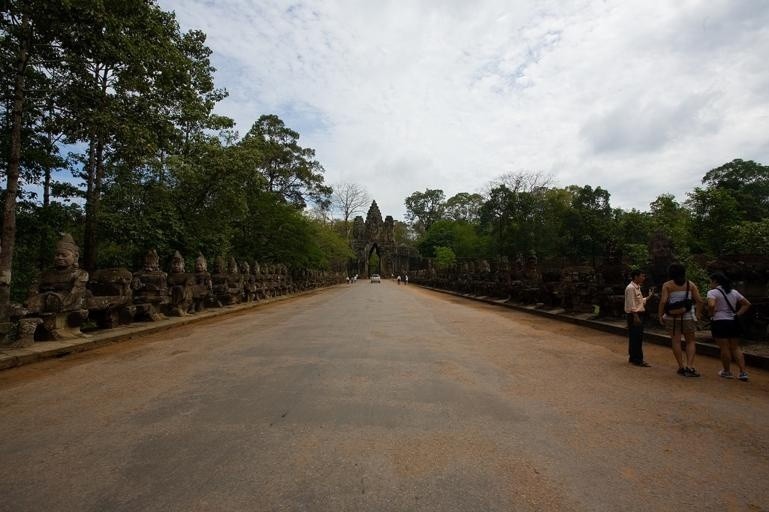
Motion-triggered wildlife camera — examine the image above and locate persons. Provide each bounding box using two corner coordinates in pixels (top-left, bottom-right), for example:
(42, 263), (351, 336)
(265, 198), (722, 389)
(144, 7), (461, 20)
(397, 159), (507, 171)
(397, 275), (401, 285)
(624, 270), (655, 368)
(346, 273), (358, 284)
(405, 274), (408, 286)
(657, 264), (704, 379)
(708, 273), (752, 381)
(648, 232), (673, 316)
(16, 232), (292, 346)
(461, 249), (543, 307)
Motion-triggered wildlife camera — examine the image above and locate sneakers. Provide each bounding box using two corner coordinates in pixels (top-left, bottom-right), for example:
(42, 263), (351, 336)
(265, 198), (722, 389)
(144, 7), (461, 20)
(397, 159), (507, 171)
(736, 371), (750, 381)
(677, 367), (700, 378)
(717, 370), (734, 378)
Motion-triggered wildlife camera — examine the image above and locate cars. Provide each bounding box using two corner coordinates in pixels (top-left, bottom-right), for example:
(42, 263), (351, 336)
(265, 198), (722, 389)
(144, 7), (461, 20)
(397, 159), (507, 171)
(370, 274), (382, 283)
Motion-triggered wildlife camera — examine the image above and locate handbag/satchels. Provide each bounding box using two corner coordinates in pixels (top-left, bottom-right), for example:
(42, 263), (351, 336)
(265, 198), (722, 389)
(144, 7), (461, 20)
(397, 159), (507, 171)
(664, 299), (694, 317)
